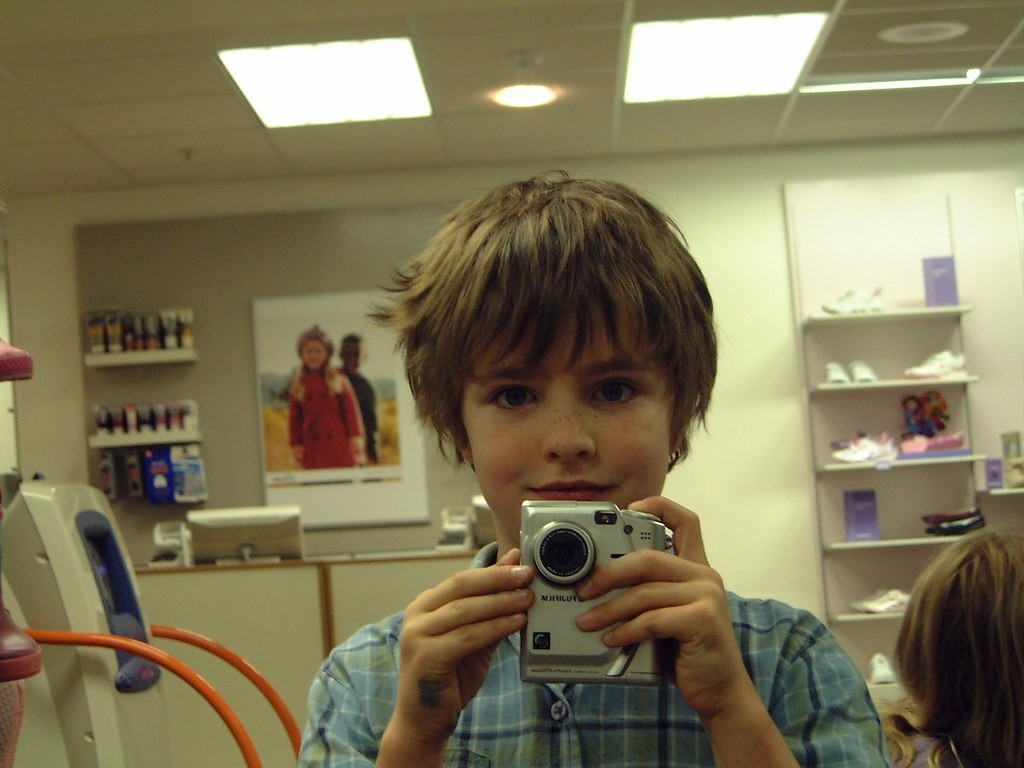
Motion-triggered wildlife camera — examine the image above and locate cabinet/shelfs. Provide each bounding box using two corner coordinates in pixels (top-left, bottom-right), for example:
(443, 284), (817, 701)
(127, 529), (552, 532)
(796, 305), (987, 713)
(83, 348), (202, 449)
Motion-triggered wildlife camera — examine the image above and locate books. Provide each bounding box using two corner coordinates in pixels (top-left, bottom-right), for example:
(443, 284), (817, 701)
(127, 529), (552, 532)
(844, 490), (878, 541)
(922, 255), (959, 308)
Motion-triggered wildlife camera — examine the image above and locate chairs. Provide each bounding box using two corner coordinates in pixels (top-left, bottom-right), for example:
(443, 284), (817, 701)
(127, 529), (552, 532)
(0, 480), (303, 768)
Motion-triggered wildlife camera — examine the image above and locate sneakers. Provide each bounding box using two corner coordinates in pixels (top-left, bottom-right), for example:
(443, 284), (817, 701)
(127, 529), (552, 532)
(822, 286), (885, 314)
(904, 350), (966, 378)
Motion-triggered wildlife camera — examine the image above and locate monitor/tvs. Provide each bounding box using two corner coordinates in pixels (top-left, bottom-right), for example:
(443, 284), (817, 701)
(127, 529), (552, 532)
(186, 505), (303, 566)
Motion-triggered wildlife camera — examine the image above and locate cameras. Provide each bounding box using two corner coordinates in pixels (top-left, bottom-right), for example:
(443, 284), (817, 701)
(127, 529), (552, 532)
(516, 501), (681, 685)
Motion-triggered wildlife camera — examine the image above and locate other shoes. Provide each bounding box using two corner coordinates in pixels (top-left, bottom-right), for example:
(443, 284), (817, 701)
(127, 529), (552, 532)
(832, 437), (898, 462)
(849, 361), (877, 382)
(848, 587), (910, 614)
(824, 362), (848, 383)
(920, 507), (984, 535)
(869, 652), (896, 684)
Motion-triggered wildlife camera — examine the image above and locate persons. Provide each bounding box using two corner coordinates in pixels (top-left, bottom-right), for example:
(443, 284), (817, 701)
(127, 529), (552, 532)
(288, 326), (379, 469)
(297, 168), (889, 768)
(902, 390), (948, 438)
(874, 528), (1024, 768)
